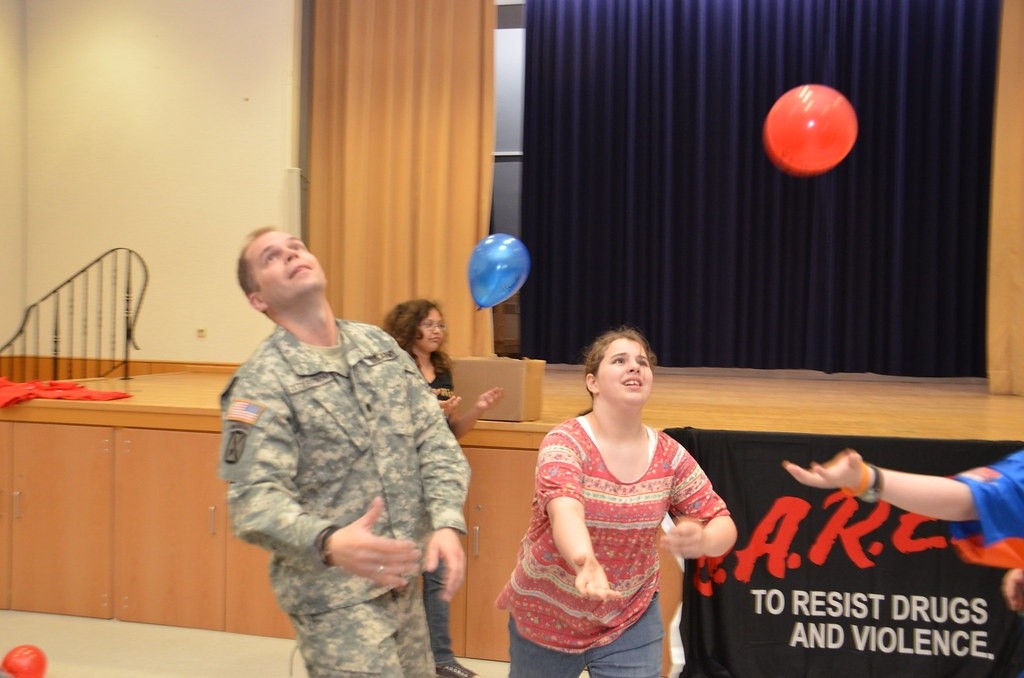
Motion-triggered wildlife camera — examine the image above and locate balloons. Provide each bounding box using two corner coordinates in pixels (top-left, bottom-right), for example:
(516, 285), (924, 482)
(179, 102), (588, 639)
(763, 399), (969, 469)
(1, 644), (48, 678)
(469, 232), (530, 311)
(763, 85), (858, 176)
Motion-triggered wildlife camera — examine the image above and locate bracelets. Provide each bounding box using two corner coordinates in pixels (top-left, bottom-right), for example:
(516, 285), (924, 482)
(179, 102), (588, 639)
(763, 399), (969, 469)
(844, 463), (869, 497)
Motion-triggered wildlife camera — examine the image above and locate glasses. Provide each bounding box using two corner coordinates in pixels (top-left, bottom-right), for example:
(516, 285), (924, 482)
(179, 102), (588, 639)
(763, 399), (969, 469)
(418, 322), (446, 330)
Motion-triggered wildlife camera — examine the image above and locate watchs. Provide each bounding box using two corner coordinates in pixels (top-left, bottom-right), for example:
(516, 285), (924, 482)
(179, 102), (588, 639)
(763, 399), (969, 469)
(856, 464), (883, 503)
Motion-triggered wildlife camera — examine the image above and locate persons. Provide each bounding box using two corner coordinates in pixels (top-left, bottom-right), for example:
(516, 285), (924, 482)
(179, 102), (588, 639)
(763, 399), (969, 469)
(219, 227), (472, 678)
(383, 299), (506, 678)
(782, 447), (1024, 610)
(495, 327), (737, 678)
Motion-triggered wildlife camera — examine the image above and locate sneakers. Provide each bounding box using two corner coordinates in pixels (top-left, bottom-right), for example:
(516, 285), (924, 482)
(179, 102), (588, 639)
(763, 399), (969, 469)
(436, 661), (481, 678)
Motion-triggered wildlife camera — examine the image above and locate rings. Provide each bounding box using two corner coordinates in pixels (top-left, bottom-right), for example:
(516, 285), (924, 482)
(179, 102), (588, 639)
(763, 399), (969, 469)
(378, 565), (385, 575)
